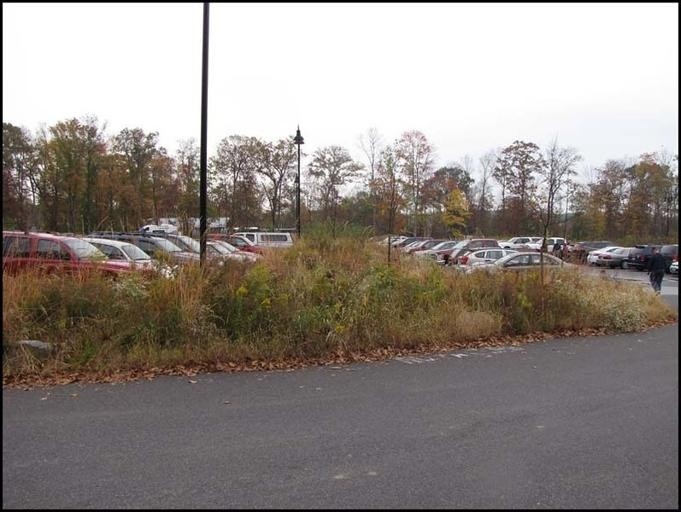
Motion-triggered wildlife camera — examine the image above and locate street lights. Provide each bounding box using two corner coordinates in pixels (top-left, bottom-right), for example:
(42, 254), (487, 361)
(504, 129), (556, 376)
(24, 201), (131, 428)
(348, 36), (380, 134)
(295, 127), (305, 237)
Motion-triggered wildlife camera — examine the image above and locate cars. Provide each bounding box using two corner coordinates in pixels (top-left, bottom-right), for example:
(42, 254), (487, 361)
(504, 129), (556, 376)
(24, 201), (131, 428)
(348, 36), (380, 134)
(378, 236), (681, 274)
(464, 251), (580, 280)
(2, 224), (295, 280)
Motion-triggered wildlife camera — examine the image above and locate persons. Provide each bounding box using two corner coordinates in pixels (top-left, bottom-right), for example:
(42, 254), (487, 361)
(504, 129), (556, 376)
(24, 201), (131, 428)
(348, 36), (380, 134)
(549, 240), (589, 266)
(646, 247), (668, 296)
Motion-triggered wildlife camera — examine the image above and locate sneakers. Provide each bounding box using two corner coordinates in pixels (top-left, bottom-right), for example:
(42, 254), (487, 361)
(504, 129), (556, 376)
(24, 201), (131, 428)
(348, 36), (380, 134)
(655, 290), (660, 296)
